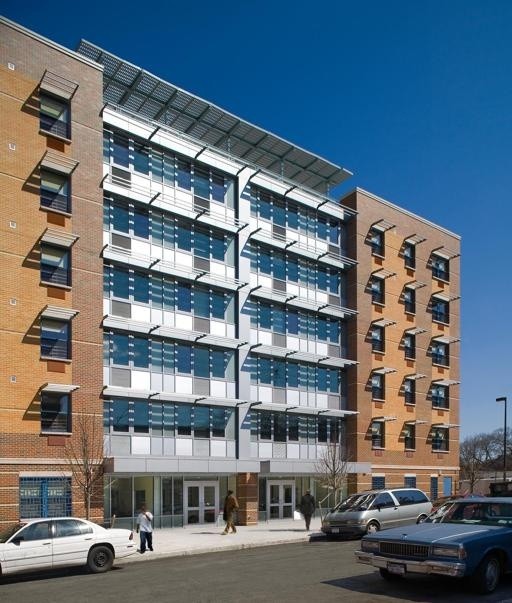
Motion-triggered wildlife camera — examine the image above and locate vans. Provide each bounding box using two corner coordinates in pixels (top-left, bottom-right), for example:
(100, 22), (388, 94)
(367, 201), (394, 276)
(317, 486), (436, 540)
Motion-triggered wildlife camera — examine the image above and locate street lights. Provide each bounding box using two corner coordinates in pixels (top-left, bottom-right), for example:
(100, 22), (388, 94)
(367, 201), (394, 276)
(496, 396), (507, 482)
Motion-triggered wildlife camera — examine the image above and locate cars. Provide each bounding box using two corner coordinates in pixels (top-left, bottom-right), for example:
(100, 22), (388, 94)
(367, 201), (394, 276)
(0, 513), (141, 582)
(352, 480), (512, 595)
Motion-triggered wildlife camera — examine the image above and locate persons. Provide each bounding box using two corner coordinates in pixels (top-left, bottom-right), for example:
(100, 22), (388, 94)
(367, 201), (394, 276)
(137, 503), (154, 554)
(221, 489), (239, 534)
(300, 489), (316, 530)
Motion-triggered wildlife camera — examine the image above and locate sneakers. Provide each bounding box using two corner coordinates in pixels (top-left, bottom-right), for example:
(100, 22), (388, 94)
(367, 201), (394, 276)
(222, 531), (237, 535)
